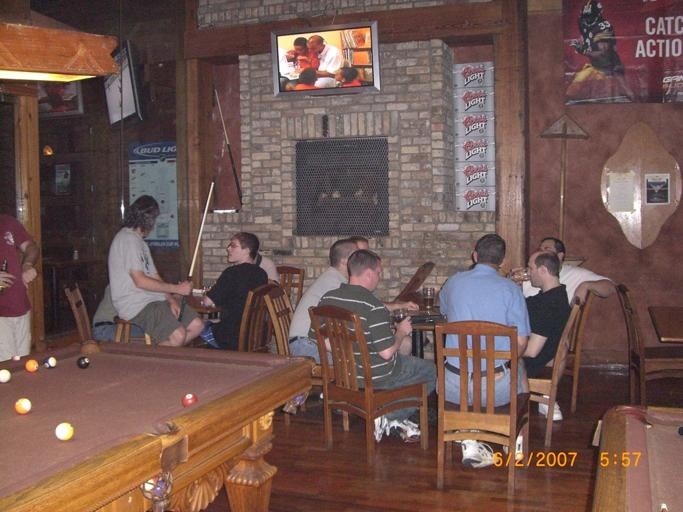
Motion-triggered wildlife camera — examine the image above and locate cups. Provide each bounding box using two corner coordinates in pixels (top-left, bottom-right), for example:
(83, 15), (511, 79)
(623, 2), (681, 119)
(423, 286), (435, 309)
(393, 308), (409, 323)
(515, 267), (529, 282)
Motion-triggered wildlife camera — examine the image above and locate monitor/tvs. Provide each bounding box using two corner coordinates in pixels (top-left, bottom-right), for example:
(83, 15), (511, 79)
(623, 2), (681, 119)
(102, 41), (144, 134)
(272, 20), (381, 102)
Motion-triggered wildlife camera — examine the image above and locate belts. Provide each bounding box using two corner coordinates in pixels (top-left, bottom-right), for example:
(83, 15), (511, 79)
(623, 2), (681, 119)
(95, 322), (112, 326)
(289, 336), (301, 343)
(444, 360), (506, 379)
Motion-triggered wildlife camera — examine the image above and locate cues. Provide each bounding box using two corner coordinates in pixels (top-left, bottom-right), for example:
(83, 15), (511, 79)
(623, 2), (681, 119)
(179, 177), (216, 322)
(212, 82), (243, 207)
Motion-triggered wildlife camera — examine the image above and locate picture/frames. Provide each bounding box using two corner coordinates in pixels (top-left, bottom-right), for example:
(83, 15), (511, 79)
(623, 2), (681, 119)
(54, 164), (71, 195)
(643, 171), (671, 206)
(37, 81), (84, 116)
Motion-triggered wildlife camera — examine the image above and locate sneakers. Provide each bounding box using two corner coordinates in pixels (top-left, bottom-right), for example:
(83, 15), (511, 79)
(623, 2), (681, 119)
(539, 395), (563, 422)
(388, 420), (424, 443)
(503, 436), (524, 460)
(374, 413), (387, 443)
(462, 440), (499, 468)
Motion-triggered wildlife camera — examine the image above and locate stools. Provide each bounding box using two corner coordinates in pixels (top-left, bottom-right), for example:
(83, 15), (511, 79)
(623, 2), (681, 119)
(115, 319), (152, 346)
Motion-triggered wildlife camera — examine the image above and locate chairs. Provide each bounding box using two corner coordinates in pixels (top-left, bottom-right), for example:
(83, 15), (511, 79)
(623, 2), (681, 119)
(567, 288), (595, 413)
(434, 321), (531, 490)
(63, 283), (93, 342)
(308, 304), (431, 453)
(527, 296), (581, 447)
(238, 284), (273, 354)
(616, 285), (683, 407)
(271, 265), (305, 336)
(263, 287), (351, 430)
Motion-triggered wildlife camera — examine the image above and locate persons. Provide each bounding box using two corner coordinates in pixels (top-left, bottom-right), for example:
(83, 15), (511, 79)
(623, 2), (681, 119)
(439, 235), (531, 469)
(563, 0), (635, 102)
(255, 252), (280, 340)
(280, 38), (363, 90)
(108, 196), (204, 348)
(513, 253), (569, 393)
(350, 237), (369, 249)
(288, 239), (419, 365)
(308, 250), (437, 443)
(196, 233), (268, 351)
(92, 283), (145, 343)
(521, 238), (617, 419)
(1, 214), (41, 362)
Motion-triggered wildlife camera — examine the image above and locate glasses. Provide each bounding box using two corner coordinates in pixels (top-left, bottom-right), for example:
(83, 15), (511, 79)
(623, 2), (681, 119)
(229, 243), (239, 248)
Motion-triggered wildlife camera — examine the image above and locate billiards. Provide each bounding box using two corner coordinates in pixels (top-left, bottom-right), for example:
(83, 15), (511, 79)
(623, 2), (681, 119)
(182, 393), (197, 407)
(15, 398), (32, 414)
(77, 357), (90, 369)
(25, 359), (39, 373)
(55, 422), (74, 440)
(43, 356), (56, 369)
(0, 369), (11, 383)
